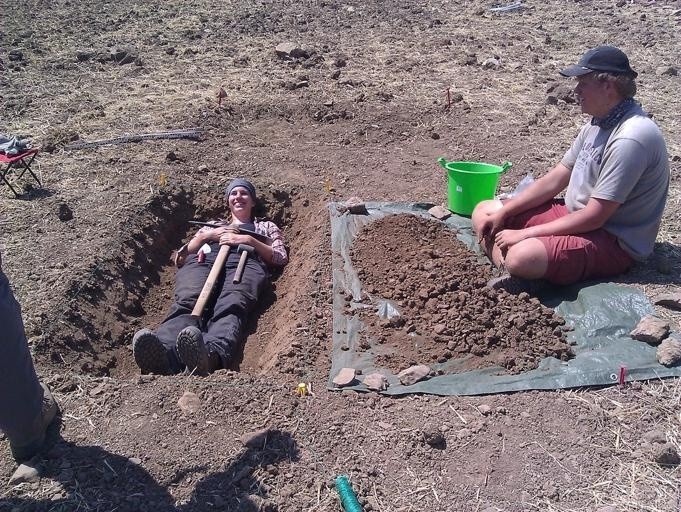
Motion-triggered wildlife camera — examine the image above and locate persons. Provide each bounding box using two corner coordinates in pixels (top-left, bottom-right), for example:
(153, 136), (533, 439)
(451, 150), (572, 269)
(469, 47), (669, 285)
(130, 179), (288, 375)
(0, 261), (61, 463)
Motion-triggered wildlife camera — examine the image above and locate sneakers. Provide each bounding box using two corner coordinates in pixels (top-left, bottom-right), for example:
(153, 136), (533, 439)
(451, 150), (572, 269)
(132, 329), (175, 375)
(10, 382), (57, 458)
(175, 326), (220, 377)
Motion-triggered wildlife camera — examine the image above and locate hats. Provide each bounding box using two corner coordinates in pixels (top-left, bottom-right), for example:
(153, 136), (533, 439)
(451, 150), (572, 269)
(226, 179), (255, 200)
(559, 46), (637, 77)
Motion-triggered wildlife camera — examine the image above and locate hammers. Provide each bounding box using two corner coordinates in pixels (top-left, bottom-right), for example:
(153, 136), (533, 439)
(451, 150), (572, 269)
(232, 244), (255, 283)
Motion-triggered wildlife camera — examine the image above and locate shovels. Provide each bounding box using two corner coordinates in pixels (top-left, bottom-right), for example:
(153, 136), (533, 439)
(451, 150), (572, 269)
(197, 243), (212, 263)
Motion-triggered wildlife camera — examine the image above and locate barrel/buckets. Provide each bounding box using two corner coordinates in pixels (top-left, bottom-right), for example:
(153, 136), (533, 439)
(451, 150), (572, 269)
(438, 157), (514, 217)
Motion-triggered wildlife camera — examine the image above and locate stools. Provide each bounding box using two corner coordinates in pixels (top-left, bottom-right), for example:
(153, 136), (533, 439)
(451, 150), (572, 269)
(0, 149), (41, 196)
